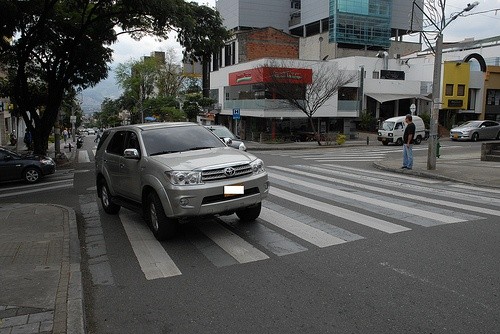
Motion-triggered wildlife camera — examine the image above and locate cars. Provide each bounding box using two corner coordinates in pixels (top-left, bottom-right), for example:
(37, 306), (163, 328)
(449, 119), (500, 142)
(204, 125), (247, 152)
(0, 146), (57, 184)
(79, 126), (100, 135)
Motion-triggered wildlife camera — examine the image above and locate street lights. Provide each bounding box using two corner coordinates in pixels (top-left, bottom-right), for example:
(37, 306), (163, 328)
(426, 1), (480, 172)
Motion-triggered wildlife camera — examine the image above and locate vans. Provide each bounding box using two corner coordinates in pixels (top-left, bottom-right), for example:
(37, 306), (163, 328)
(377, 115), (427, 146)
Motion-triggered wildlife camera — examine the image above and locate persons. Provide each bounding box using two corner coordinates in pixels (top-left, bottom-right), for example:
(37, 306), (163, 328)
(401, 113), (416, 170)
(23, 128), (32, 150)
(63, 128), (68, 141)
(94, 127), (105, 144)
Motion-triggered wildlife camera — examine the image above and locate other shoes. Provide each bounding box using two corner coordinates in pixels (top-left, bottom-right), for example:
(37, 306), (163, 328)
(402, 166), (412, 170)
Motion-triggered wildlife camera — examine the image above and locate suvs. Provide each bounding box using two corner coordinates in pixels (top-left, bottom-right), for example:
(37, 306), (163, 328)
(94, 122), (271, 238)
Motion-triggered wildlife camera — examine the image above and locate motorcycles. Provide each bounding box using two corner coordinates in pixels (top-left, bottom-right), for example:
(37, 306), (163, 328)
(75, 135), (86, 149)
